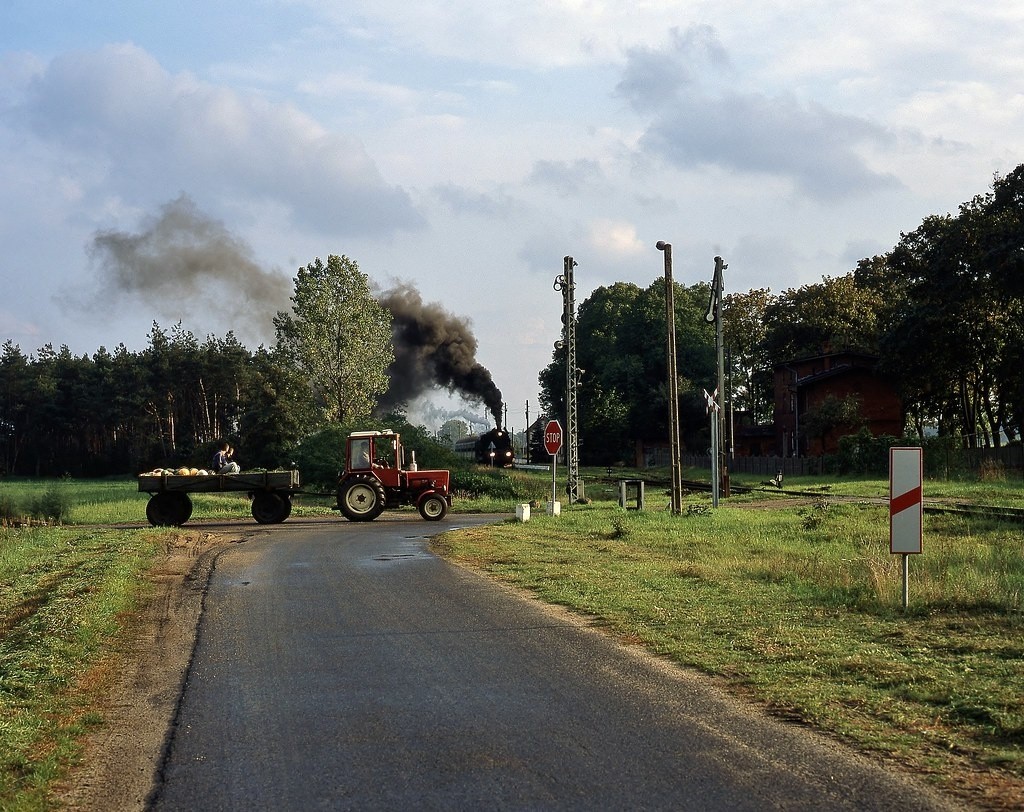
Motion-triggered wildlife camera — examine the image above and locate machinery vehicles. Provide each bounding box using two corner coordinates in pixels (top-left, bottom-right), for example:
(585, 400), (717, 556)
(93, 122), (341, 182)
(334, 430), (451, 521)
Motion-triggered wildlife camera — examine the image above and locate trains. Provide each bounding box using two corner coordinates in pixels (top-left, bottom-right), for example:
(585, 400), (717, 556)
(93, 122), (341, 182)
(453, 428), (515, 469)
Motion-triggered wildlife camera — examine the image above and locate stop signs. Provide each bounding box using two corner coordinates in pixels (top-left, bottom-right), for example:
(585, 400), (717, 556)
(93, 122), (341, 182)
(542, 419), (563, 455)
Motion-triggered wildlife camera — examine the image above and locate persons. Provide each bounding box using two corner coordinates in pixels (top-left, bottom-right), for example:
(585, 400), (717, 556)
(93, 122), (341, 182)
(224, 446), (240, 472)
(212, 443), (240, 474)
(359, 442), (389, 470)
